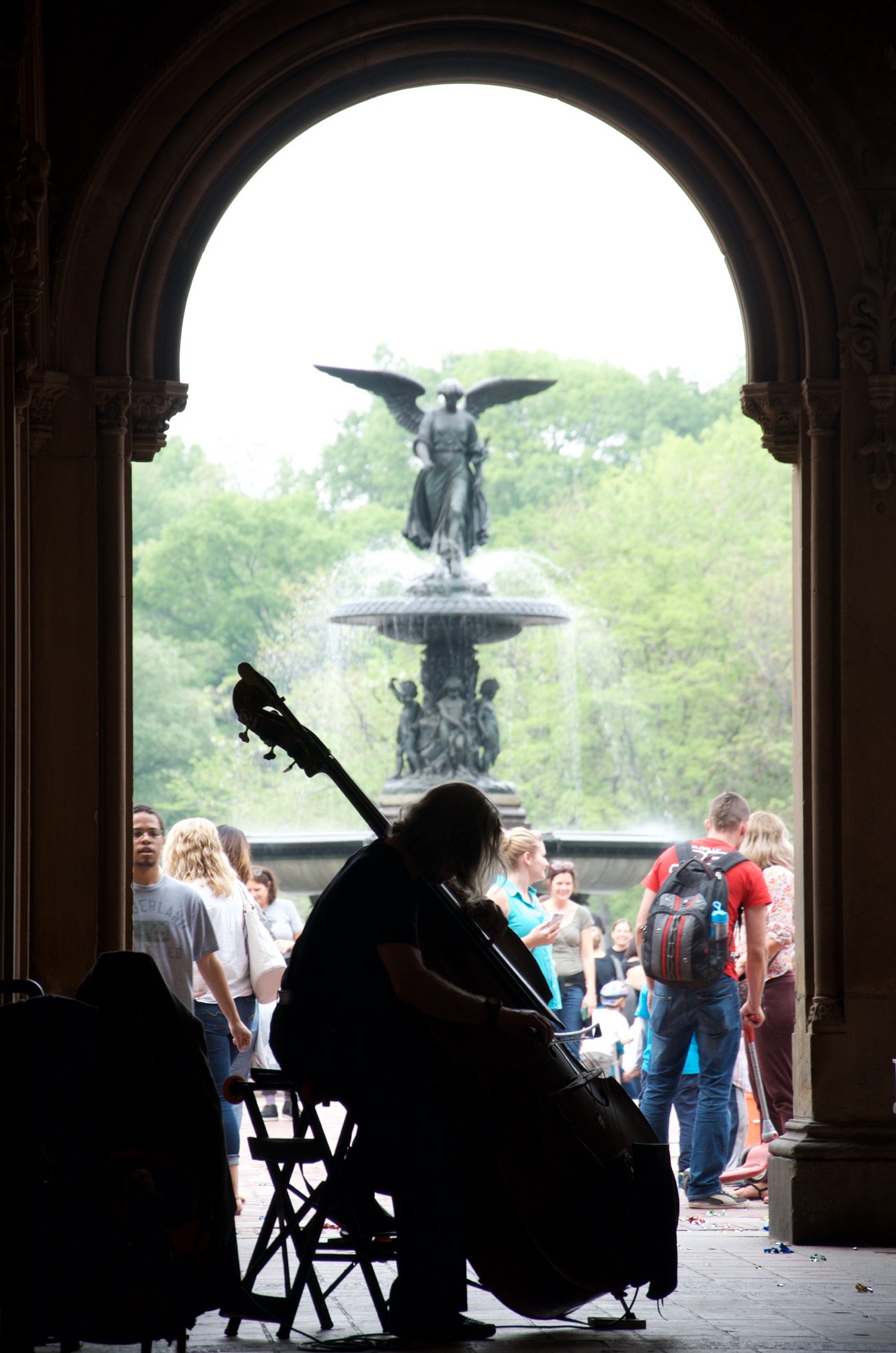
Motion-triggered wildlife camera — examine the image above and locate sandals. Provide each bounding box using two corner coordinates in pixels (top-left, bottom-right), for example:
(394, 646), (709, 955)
(729, 1183), (768, 1200)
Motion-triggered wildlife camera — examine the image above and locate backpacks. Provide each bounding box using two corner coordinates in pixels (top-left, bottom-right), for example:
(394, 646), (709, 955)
(641, 840), (752, 990)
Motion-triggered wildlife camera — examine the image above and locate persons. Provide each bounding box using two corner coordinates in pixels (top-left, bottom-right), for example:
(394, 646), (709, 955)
(388, 675), (500, 781)
(268, 781), (558, 1336)
(414, 380), (488, 580)
(130, 792), (797, 1206)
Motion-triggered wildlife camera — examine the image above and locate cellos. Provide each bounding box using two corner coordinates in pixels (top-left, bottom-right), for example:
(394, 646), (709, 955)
(228, 660), (686, 1323)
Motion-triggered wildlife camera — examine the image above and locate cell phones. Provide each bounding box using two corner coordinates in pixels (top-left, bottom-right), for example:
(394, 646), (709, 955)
(548, 913), (563, 928)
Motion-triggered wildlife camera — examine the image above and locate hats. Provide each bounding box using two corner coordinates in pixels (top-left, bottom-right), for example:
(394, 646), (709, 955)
(591, 914), (605, 936)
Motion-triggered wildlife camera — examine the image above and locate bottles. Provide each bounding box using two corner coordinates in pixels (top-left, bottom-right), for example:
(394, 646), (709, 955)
(710, 901), (729, 941)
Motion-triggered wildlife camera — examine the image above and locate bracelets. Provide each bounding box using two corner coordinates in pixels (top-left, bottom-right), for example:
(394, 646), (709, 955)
(480, 998), (502, 1023)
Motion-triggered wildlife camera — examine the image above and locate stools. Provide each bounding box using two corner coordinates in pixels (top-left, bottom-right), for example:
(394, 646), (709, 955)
(223, 1078), (389, 1341)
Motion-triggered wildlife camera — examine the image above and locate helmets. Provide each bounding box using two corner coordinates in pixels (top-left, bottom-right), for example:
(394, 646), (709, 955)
(599, 980), (630, 1006)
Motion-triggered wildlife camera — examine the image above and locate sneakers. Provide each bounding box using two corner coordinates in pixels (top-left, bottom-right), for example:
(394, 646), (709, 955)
(688, 1190), (750, 1209)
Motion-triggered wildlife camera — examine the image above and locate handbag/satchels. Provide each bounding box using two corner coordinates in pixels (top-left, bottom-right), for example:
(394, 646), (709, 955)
(239, 880), (287, 1005)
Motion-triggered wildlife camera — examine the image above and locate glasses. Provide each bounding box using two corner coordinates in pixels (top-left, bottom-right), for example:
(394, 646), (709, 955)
(548, 860), (574, 870)
(132, 828), (165, 839)
(252, 869), (269, 877)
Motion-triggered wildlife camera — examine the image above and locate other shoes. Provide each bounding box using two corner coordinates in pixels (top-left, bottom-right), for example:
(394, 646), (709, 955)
(233, 1196), (245, 1215)
(259, 1104), (278, 1121)
(281, 1101), (302, 1121)
(308, 1181), (397, 1235)
(383, 1301), (496, 1339)
(677, 1172), (684, 1188)
